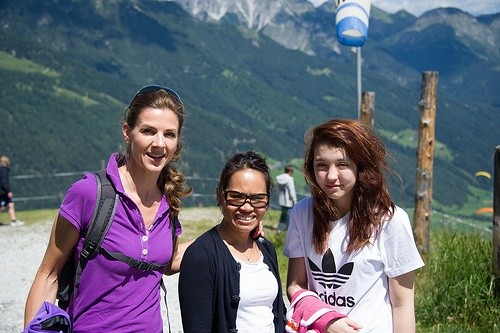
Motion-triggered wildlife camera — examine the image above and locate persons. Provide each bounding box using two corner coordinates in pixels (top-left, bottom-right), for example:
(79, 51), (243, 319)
(282, 118), (425, 333)
(0, 156), (25, 227)
(275, 165), (297, 231)
(23, 84), (196, 333)
(177, 150), (287, 333)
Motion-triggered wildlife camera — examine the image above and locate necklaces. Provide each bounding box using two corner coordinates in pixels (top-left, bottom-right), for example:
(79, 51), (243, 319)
(235, 242), (257, 262)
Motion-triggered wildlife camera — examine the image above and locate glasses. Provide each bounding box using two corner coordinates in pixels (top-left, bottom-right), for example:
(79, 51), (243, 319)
(129, 85), (183, 109)
(220, 187), (272, 210)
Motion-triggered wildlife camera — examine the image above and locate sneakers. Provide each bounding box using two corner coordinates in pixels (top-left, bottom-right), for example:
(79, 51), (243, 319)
(10, 219), (26, 227)
